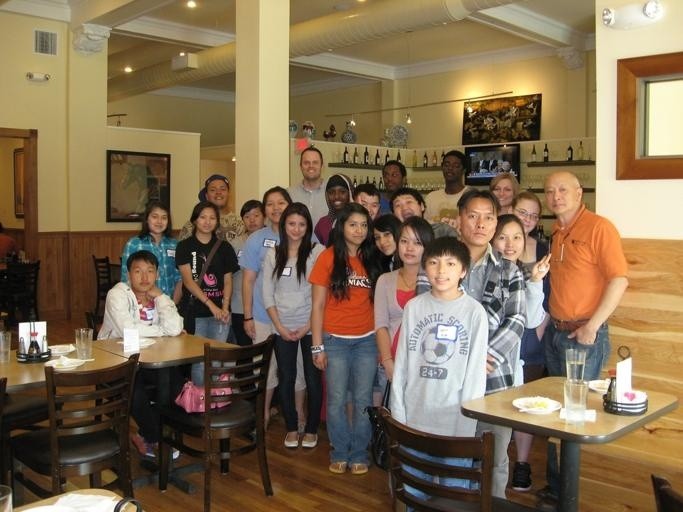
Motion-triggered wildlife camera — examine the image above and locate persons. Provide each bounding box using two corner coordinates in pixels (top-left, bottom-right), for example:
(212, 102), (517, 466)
(489, 173), (520, 216)
(536, 172), (629, 502)
(175, 201), (240, 386)
(361, 214), (403, 303)
(97, 251), (184, 458)
(285, 147), (329, 244)
(176, 174), (247, 242)
(121, 199), (184, 306)
(389, 188), (460, 239)
(378, 160), (407, 219)
(352, 183), (381, 222)
(508, 192), (551, 492)
(260, 202), (326, 447)
(423, 150), (472, 235)
(373, 215), (434, 407)
(314, 174), (355, 248)
(390, 236), (488, 512)
(457, 190), (528, 500)
(489, 214), (551, 330)
(310, 203), (391, 475)
(238, 186), (319, 434)
(229, 199), (266, 441)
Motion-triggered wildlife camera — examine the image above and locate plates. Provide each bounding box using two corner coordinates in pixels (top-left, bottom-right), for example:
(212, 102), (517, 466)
(512, 396), (560, 413)
(585, 380), (614, 393)
(136, 338), (156, 347)
(44, 359), (85, 370)
(49, 345), (74, 355)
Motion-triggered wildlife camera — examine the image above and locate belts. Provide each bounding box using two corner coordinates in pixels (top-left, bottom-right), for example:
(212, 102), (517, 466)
(549, 318), (588, 332)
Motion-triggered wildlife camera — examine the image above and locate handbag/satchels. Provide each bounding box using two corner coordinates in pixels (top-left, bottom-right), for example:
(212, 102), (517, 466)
(174, 373), (233, 414)
(363, 380), (391, 473)
(183, 238), (222, 335)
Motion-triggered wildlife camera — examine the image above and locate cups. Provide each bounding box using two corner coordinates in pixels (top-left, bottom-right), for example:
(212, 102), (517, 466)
(75, 329), (92, 360)
(565, 349), (585, 381)
(565, 381), (589, 426)
(1, 331), (12, 363)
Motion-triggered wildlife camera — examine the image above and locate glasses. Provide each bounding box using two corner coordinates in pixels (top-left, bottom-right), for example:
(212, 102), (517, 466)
(549, 235), (564, 263)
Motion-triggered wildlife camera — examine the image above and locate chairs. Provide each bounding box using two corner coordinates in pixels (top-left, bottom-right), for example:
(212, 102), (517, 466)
(155, 333), (277, 509)
(92, 256), (121, 314)
(366, 404), (495, 512)
(0, 259), (39, 328)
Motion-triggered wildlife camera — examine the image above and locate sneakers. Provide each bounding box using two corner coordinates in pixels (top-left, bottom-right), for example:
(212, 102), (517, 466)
(130, 433), (156, 459)
(512, 461), (534, 492)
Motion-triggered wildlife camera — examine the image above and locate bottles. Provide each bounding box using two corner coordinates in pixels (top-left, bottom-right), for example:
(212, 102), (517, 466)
(28, 333), (41, 354)
(611, 380), (616, 402)
(379, 177), (383, 189)
(373, 177), (376, 186)
(354, 147), (358, 164)
(385, 149), (389, 162)
(441, 152), (445, 165)
(376, 149), (380, 165)
(353, 175), (357, 188)
(578, 141), (584, 159)
(433, 151), (436, 166)
(537, 225), (541, 239)
(366, 176), (369, 184)
(360, 175), (363, 185)
(344, 146), (349, 164)
(397, 150), (401, 163)
(541, 224), (544, 238)
(413, 150), (417, 167)
(42, 336), (49, 358)
(567, 145), (573, 160)
(424, 151), (427, 167)
(531, 144), (536, 161)
(364, 147), (369, 164)
(18, 337), (26, 361)
(544, 144), (548, 161)
(606, 378), (615, 402)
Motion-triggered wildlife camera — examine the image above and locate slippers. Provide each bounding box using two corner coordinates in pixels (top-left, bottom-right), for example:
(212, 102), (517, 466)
(350, 463), (369, 475)
(329, 461), (348, 474)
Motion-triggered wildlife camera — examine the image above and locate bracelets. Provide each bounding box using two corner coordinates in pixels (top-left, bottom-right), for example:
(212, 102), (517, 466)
(377, 356), (394, 366)
(201, 297), (209, 304)
(243, 317), (254, 322)
(309, 344), (325, 354)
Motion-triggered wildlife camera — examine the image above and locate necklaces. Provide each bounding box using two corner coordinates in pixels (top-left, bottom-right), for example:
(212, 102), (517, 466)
(398, 270), (417, 291)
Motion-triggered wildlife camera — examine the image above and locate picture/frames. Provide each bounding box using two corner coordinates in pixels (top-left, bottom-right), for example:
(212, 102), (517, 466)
(12, 148), (29, 219)
(106, 148), (172, 225)
(462, 93), (544, 145)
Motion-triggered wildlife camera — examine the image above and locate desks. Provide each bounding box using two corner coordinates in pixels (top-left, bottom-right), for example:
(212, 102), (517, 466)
(462, 374), (678, 510)
(0, 342), (136, 511)
(92, 330), (242, 493)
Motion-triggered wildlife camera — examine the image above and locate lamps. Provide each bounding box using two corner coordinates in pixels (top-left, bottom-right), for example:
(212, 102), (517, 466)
(406, 30), (414, 124)
(349, 114), (355, 127)
(467, 100), (473, 116)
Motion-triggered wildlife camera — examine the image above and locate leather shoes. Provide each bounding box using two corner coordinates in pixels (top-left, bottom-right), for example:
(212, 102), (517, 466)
(302, 433), (319, 448)
(283, 431), (300, 449)
